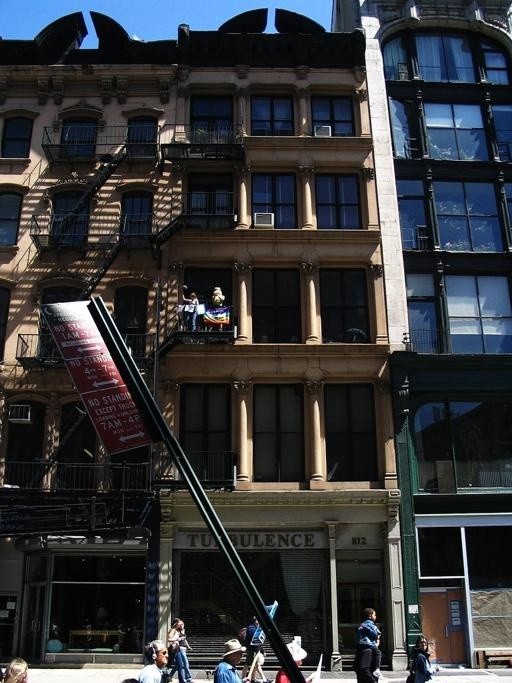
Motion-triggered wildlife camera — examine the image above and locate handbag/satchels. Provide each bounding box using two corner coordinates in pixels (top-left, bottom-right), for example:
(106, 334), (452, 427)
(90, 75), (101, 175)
(168, 641), (180, 654)
(406, 674), (415, 683)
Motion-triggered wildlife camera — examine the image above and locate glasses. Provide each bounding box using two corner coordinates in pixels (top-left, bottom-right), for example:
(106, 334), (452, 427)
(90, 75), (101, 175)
(161, 651), (166, 655)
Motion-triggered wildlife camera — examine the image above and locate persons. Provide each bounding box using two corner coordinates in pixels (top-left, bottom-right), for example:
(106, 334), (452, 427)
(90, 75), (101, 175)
(353, 634), (383, 683)
(412, 635), (440, 683)
(357, 607), (385, 679)
(207, 285), (227, 332)
(181, 293), (201, 331)
(121, 608), (307, 683)
(4, 658), (28, 683)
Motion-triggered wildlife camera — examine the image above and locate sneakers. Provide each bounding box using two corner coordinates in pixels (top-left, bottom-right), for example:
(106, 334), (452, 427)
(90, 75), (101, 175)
(373, 670), (383, 680)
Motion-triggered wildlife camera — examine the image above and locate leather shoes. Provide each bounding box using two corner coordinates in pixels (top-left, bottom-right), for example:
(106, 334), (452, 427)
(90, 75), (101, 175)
(252, 679), (261, 682)
(263, 678), (273, 682)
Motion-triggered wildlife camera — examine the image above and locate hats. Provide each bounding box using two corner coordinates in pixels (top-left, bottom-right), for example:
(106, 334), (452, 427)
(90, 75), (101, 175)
(285, 643), (307, 661)
(222, 638), (247, 657)
